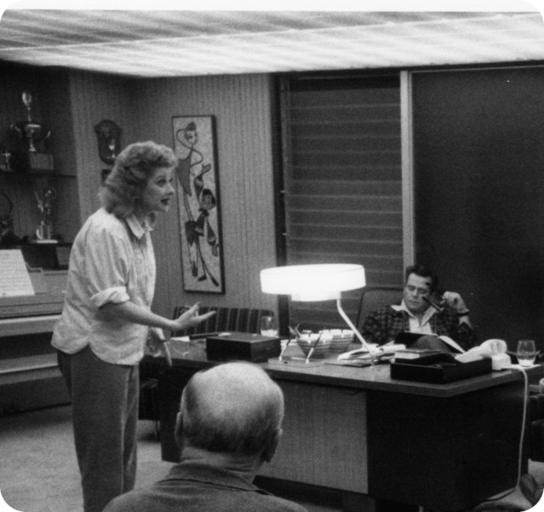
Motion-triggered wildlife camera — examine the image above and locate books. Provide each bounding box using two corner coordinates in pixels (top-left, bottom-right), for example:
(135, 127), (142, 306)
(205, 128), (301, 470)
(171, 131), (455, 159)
(337, 346), (375, 367)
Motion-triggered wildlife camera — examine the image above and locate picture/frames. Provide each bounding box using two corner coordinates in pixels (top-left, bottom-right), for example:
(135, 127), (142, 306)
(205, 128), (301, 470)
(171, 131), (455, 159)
(170, 114), (227, 295)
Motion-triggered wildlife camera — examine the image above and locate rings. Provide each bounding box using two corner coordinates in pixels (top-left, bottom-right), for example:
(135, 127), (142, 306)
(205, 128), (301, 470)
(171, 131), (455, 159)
(159, 339), (167, 345)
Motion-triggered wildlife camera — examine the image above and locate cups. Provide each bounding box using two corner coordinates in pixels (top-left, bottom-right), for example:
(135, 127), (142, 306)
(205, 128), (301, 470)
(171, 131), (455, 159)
(514, 340), (537, 367)
(260, 316), (279, 337)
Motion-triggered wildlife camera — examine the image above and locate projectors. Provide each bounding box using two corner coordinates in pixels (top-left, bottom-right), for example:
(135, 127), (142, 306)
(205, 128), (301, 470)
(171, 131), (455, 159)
(206, 329), (282, 363)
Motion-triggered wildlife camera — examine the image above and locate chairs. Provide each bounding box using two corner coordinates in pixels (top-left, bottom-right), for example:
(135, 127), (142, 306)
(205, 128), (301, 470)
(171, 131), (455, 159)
(352, 285), (441, 344)
(139, 306), (275, 442)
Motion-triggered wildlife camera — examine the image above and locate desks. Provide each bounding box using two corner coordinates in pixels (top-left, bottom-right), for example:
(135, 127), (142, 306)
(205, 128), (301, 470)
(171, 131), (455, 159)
(145, 330), (544, 512)
(0, 314), (64, 386)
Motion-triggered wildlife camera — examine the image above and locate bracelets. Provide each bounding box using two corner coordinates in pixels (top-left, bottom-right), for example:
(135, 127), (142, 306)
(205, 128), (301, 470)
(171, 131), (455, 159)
(458, 308), (470, 317)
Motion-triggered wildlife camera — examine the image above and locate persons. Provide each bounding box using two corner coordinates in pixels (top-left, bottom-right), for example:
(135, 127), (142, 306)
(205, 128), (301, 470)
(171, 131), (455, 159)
(355, 263), (478, 354)
(51, 141), (217, 512)
(101, 360), (308, 511)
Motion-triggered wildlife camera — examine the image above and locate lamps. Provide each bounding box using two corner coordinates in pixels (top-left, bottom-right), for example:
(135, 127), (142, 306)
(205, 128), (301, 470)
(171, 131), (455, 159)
(259, 262), (381, 373)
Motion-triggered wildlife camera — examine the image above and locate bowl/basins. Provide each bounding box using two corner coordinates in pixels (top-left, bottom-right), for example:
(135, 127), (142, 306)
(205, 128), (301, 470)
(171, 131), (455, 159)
(297, 333), (332, 358)
(318, 329), (355, 353)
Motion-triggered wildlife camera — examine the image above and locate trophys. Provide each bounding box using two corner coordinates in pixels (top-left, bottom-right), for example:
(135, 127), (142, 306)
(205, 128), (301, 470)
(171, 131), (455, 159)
(29, 184), (61, 245)
(10, 91), (53, 153)
